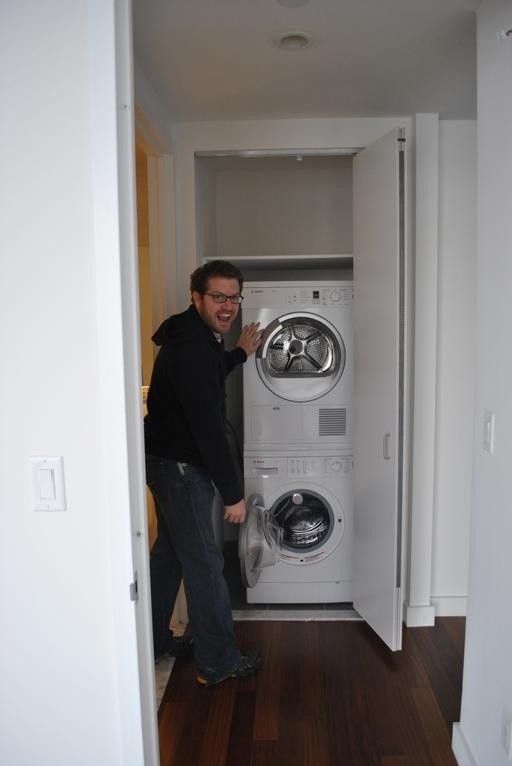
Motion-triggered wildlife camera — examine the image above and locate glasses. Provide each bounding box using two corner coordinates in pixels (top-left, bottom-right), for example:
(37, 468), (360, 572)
(205, 293), (243, 304)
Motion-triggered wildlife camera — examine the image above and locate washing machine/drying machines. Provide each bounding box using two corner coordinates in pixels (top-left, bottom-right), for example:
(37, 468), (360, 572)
(238, 452), (357, 604)
(237, 279), (356, 451)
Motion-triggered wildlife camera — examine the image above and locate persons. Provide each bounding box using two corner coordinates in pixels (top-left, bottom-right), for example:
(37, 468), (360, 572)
(144, 260), (266, 686)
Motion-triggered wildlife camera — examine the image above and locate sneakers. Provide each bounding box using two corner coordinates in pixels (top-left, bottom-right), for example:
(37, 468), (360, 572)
(155, 629), (193, 663)
(197, 654), (262, 687)
